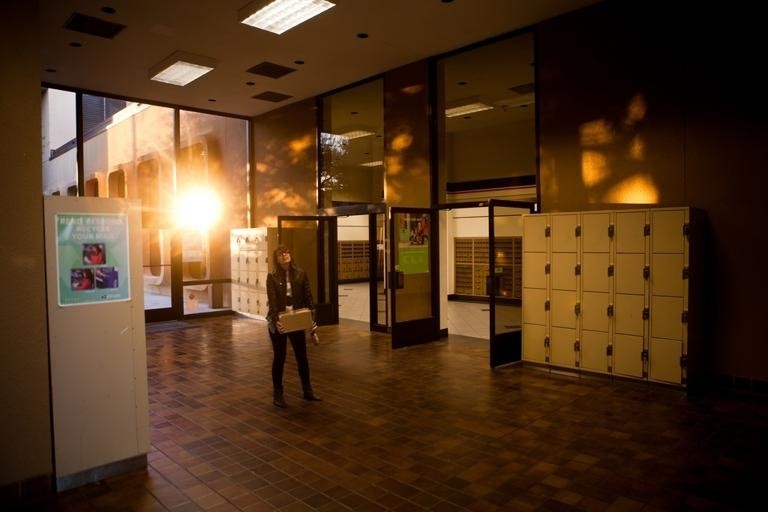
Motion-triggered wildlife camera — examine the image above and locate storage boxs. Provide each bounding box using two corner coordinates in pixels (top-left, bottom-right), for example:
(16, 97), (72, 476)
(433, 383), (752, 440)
(276, 308), (315, 334)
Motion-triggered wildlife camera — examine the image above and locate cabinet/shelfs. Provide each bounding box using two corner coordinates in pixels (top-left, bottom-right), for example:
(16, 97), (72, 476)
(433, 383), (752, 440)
(41, 190), (151, 491)
(230, 229), (318, 322)
(452, 233), (521, 296)
(338, 240), (370, 283)
(522, 206), (707, 393)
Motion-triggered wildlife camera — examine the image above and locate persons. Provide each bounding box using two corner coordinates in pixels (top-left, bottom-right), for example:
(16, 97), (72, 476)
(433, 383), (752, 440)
(266, 246), (323, 408)
(84, 244), (105, 264)
(73, 269), (94, 289)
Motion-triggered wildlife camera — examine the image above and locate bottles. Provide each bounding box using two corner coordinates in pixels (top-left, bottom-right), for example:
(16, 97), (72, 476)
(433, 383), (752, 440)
(311, 329), (320, 347)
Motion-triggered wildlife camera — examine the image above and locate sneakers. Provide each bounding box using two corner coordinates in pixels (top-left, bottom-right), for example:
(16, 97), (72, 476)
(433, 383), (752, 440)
(302, 387), (324, 402)
(273, 390), (288, 409)
